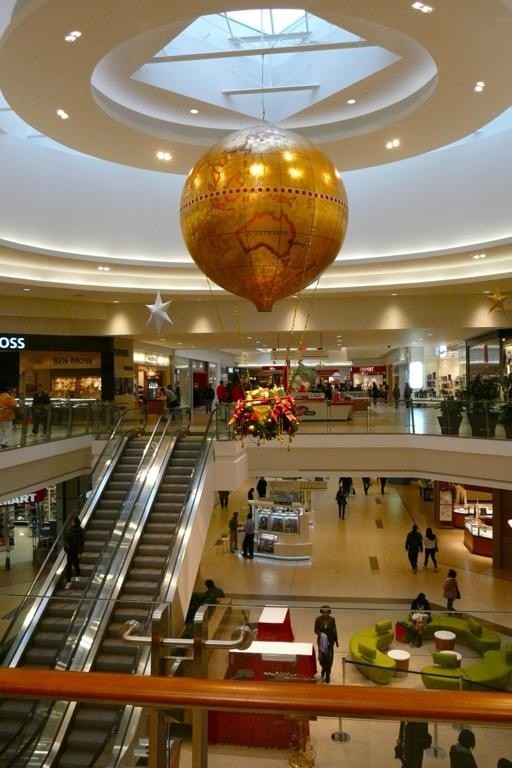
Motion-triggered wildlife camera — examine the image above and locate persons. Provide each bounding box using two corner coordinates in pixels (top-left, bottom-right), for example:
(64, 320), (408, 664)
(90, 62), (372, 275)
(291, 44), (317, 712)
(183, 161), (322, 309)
(449, 729), (480, 768)
(168, 384), (177, 395)
(337, 477), (353, 498)
(160, 388), (177, 420)
(334, 486), (347, 520)
(62, 515), (86, 591)
(256, 475), (269, 498)
(318, 380), (412, 409)
(395, 719), (429, 768)
(376, 477), (388, 496)
(27, 384), (51, 438)
(1, 386), (19, 448)
(422, 526), (440, 572)
(362, 477), (371, 496)
(204, 578), (225, 598)
(405, 524), (424, 573)
(218, 490), (230, 509)
(247, 487), (255, 514)
(313, 604), (340, 685)
(203, 375), (256, 421)
(410, 591), (433, 649)
(241, 513), (255, 560)
(228, 512), (242, 554)
(442, 568), (463, 619)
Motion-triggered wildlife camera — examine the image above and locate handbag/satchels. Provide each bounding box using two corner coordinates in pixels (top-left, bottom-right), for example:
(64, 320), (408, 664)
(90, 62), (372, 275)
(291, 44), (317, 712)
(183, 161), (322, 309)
(457, 591), (461, 599)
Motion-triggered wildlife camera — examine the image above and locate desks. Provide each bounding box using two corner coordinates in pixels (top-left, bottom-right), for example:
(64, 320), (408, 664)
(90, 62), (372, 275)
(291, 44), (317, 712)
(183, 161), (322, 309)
(208, 602), (317, 749)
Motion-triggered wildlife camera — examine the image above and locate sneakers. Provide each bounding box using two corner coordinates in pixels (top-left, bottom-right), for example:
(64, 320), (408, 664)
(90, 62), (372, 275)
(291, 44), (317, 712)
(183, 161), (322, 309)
(321, 668), (331, 683)
(65, 574), (81, 590)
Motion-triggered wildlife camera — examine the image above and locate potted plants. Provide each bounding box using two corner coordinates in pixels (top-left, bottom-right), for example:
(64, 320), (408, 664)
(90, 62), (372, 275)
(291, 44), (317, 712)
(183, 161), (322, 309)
(436, 373), (512, 439)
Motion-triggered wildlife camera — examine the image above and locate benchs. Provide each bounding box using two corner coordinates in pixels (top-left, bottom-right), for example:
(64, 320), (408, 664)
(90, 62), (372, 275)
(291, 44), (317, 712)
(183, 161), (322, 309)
(350, 612), (511, 691)
